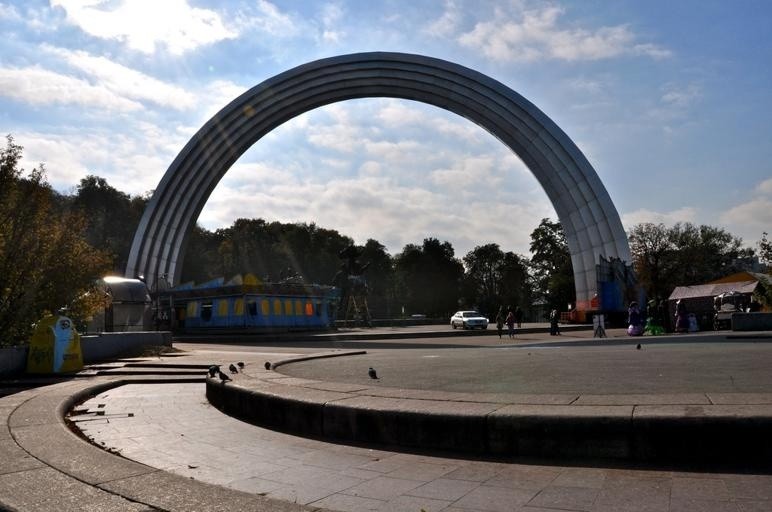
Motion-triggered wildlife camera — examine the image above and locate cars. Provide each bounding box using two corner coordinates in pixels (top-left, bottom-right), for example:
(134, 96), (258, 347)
(450, 311), (490, 330)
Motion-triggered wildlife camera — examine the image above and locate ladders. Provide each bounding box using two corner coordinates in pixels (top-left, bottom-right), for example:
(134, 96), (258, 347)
(343, 294), (373, 328)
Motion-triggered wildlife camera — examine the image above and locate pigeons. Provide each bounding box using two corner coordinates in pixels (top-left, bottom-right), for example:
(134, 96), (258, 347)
(368, 367), (380, 379)
(264, 361), (271, 371)
(637, 343), (641, 350)
(209, 362), (244, 382)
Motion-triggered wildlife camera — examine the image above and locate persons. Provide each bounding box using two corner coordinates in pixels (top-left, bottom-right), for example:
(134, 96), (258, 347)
(674, 300), (690, 335)
(627, 300), (642, 335)
(495, 312), (506, 339)
(499, 305), (522, 328)
(506, 312), (516, 339)
(550, 310), (561, 335)
